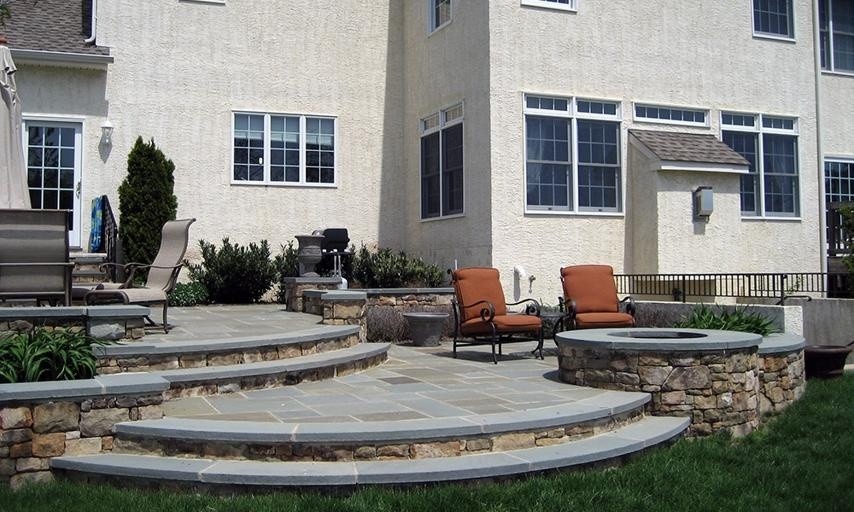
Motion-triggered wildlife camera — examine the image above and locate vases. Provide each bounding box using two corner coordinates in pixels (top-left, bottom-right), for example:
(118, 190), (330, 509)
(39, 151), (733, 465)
(806, 341), (853, 377)
(403, 309), (451, 347)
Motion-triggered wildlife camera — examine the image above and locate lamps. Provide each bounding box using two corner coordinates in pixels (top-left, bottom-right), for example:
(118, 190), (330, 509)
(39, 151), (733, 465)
(690, 182), (714, 220)
(100, 112), (115, 144)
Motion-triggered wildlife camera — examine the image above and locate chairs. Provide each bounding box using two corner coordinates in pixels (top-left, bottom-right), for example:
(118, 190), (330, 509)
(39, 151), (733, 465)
(447, 262), (545, 361)
(555, 262), (639, 333)
(96, 213), (196, 332)
(0, 206), (76, 307)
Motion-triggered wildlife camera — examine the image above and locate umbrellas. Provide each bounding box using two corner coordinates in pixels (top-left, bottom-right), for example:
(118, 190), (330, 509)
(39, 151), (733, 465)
(0, 38), (33, 210)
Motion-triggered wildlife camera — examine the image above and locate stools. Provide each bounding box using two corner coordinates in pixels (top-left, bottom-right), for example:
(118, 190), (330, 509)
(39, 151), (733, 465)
(81, 286), (170, 334)
